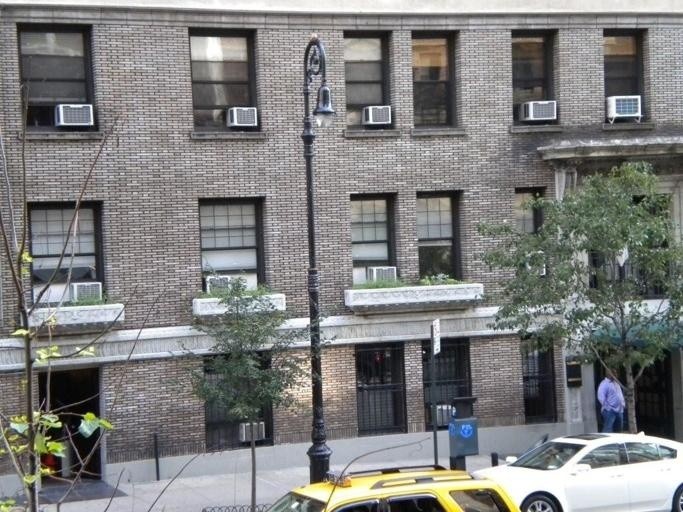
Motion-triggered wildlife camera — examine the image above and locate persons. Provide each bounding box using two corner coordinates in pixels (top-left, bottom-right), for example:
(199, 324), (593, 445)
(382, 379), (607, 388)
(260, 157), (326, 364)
(596, 366), (626, 433)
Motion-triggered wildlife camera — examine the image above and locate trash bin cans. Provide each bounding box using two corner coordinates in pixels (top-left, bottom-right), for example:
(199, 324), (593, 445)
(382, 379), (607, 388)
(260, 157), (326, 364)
(448, 397), (478, 457)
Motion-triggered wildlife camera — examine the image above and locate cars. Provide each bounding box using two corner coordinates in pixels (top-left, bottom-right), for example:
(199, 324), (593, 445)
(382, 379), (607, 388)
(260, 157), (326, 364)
(469, 428), (681, 512)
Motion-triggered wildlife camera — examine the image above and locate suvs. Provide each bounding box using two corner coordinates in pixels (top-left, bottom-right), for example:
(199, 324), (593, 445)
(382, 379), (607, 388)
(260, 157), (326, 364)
(260, 462), (522, 512)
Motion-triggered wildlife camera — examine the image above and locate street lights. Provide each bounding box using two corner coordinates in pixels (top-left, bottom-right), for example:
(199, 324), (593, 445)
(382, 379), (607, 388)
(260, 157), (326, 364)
(299, 32), (340, 485)
